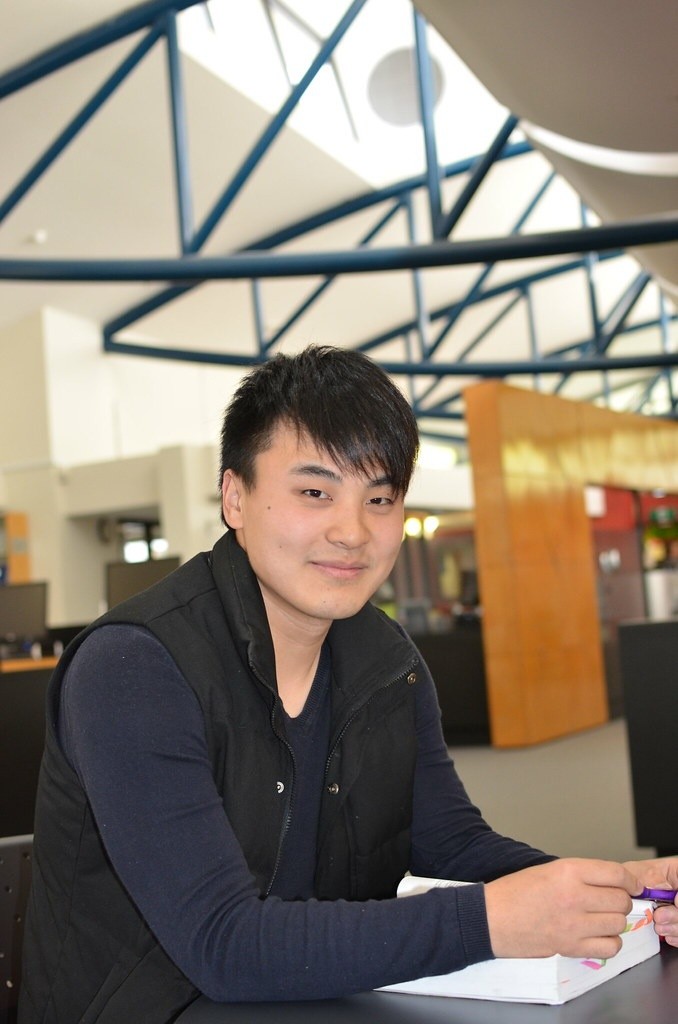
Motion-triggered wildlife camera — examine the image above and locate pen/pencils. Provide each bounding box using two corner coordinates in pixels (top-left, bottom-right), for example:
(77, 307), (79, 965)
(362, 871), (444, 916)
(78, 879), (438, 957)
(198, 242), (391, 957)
(629, 887), (678, 905)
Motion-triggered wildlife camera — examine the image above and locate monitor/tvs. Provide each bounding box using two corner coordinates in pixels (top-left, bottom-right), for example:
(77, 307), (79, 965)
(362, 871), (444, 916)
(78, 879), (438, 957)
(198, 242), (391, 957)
(0, 582), (48, 660)
(107, 557), (180, 613)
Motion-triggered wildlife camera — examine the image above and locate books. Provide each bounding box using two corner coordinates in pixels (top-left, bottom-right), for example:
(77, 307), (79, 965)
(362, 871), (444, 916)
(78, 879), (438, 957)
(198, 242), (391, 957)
(374, 875), (660, 1005)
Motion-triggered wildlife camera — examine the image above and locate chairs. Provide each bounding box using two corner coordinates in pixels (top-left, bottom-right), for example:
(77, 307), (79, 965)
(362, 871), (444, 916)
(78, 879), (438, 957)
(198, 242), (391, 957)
(0, 833), (35, 1024)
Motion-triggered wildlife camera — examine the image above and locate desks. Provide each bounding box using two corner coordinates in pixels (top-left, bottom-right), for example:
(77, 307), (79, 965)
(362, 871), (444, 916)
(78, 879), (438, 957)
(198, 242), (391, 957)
(177, 937), (678, 1024)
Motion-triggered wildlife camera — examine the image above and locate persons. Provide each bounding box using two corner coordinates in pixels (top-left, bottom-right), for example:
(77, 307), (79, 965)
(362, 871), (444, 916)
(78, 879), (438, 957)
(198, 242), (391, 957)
(452, 570), (482, 628)
(17, 345), (678, 1024)
